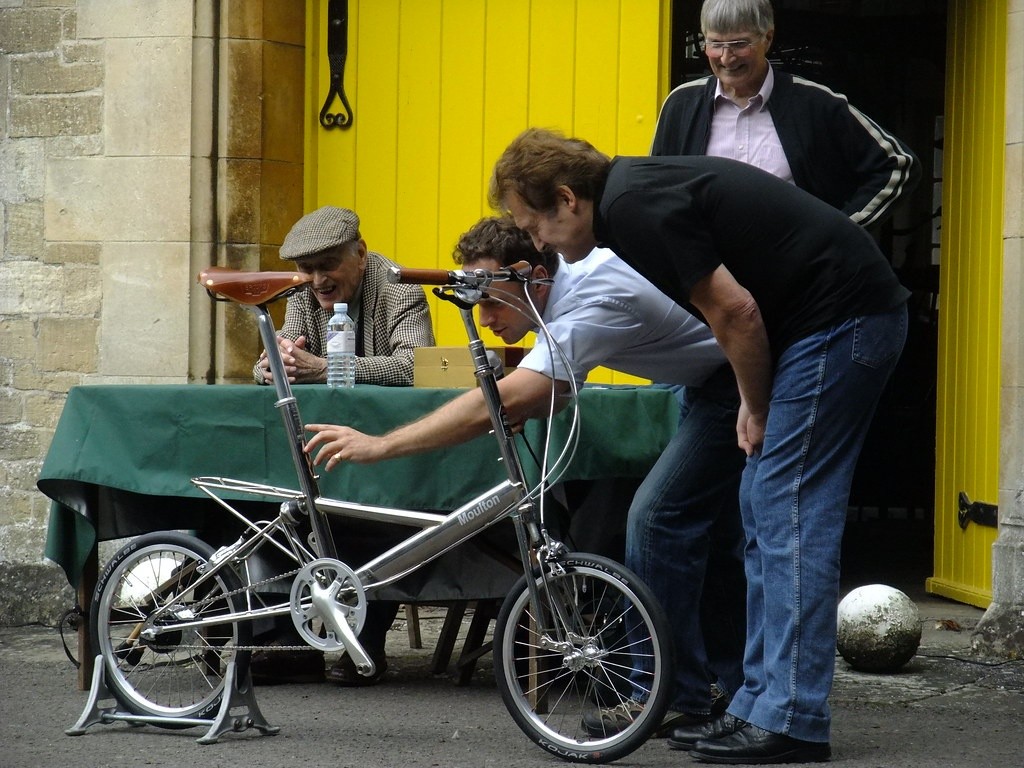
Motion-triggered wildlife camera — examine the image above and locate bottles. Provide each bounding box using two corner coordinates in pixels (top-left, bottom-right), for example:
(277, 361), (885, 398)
(326, 303), (356, 389)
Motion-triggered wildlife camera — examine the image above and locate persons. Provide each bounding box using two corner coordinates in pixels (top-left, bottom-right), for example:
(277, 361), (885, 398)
(651, 1), (922, 229)
(252, 207), (437, 386)
(485, 129), (913, 763)
(301, 217), (747, 737)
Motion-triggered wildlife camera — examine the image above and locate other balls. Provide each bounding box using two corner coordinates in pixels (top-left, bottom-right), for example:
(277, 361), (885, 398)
(838, 583), (921, 672)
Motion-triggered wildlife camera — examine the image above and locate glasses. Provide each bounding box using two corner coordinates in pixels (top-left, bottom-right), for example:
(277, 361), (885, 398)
(704, 33), (763, 58)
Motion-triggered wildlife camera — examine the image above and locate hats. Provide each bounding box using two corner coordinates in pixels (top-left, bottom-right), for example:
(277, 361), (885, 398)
(279, 207), (362, 261)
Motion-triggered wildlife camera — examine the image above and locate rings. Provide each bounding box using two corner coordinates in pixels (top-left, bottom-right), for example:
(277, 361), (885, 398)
(334, 453), (342, 462)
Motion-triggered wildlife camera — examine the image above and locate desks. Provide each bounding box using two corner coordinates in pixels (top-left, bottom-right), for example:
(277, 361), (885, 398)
(36, 385), (686, 714)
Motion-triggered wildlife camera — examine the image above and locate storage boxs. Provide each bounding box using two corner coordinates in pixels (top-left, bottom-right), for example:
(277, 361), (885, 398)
(414, 346), (531, 388)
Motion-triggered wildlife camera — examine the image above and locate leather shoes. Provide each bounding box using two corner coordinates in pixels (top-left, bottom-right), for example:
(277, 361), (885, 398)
(668, 712), (747, 749)
(689, 723), (831, 764)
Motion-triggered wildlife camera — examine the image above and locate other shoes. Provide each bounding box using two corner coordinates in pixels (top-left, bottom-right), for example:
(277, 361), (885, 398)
(237, 641), (326, 684)
(327, 651), (388, 685)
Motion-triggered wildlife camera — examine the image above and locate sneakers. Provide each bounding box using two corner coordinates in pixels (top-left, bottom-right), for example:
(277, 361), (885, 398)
(582, 700), (645, 738)
(657, 684), (730, 737)
